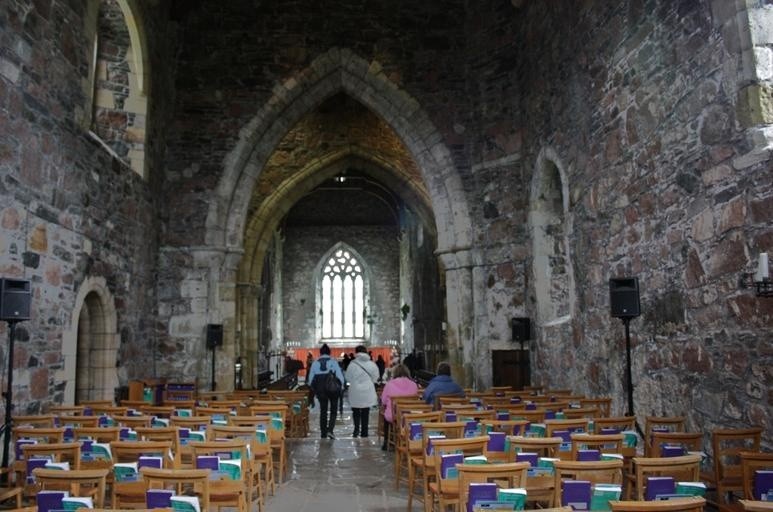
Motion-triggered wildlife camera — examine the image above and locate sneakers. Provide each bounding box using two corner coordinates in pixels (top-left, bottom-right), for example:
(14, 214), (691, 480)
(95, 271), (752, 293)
(326, 432), (336, 438)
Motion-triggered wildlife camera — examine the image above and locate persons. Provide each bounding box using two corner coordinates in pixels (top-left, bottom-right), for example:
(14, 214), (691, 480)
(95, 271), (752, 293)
(369, 350), (373, 360)
(306, 344), (345, 441)
(306, 351), (312, 383)
(386, 365), (393, 381)
(403, 353), (417, 378)
(395, 359), (401, 376)
(375, 355), (386, 382)
(342, 353), (350, 390)
(349, 352), (355, 361)
(380, 364), (418, 451)
(391, 363), (397, 377)
(345, 345), (379, 439)
(423, 362), (464, 411)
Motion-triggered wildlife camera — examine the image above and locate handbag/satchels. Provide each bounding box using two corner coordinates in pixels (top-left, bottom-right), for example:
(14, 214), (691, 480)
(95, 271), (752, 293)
(325, 364), (340, 397)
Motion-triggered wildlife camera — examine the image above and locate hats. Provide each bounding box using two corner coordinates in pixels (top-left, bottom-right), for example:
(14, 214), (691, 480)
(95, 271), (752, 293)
(320, 343), (330, 355)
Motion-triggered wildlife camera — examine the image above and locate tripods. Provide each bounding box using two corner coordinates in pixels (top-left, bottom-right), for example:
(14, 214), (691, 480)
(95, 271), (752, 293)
(618, 321), (645, 437)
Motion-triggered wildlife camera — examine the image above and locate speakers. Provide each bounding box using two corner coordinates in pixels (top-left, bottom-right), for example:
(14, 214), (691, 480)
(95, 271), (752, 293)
(2, 277), (30, 320)
(512, 317), (531, 341)
(609, 276), (639, 319)
(207, 324), (222, 347)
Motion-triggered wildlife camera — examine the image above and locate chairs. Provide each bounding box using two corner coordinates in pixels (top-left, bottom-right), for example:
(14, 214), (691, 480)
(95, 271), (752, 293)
(378, 387), (773, 512)
(0, 389), (311, 512)
